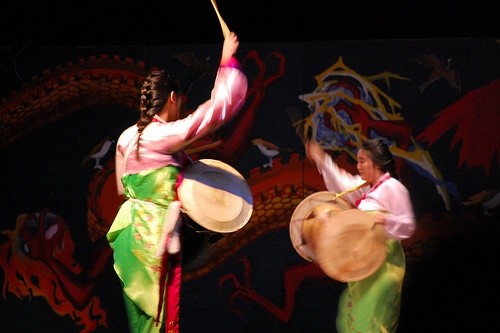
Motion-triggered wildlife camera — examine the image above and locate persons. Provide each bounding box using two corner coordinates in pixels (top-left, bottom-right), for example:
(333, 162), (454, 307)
(106, 32), (249, 333)
(304, 136), (415, 333)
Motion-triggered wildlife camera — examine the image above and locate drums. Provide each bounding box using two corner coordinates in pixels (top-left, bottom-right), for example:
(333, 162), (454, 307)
(176, 158), (253, 232)
(290, 192), (387, 282)
